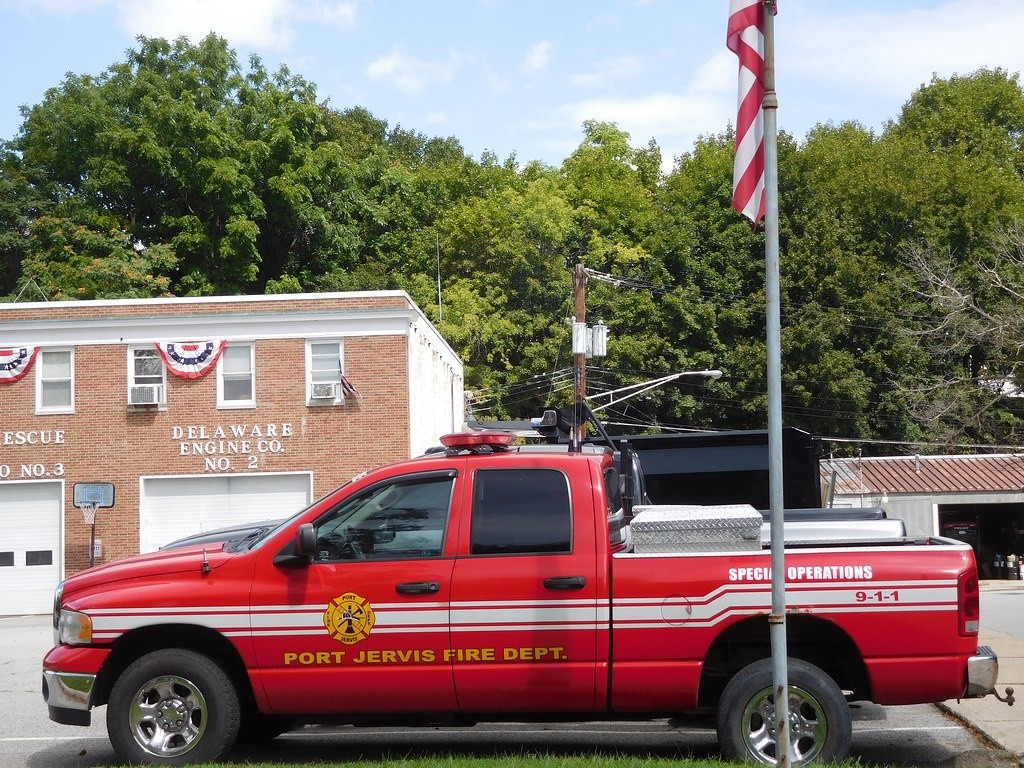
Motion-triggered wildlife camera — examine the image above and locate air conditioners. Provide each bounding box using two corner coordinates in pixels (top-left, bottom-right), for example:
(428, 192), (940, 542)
(310, 383), (338, 399)
(128, 385), (161, 406)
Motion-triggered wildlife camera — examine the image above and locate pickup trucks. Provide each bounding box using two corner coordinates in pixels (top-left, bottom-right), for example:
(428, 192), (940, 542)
(42, 428), (999, 768)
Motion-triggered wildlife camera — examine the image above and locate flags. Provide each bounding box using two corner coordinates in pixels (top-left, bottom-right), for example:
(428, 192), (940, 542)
(726, 0), (765, 230)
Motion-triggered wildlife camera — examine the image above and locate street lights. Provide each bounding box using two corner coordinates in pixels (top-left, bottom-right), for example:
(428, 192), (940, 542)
(574, 368), (723, 439)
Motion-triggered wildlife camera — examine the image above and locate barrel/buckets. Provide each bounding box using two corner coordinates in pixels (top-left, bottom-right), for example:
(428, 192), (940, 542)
(994, 561), (1007, 579)
(1007, 556), (1019, 561)
(995, 555), (1006, 561)
(1007, 561), (1020, 580)
(1019, 556), (1024, 580)
